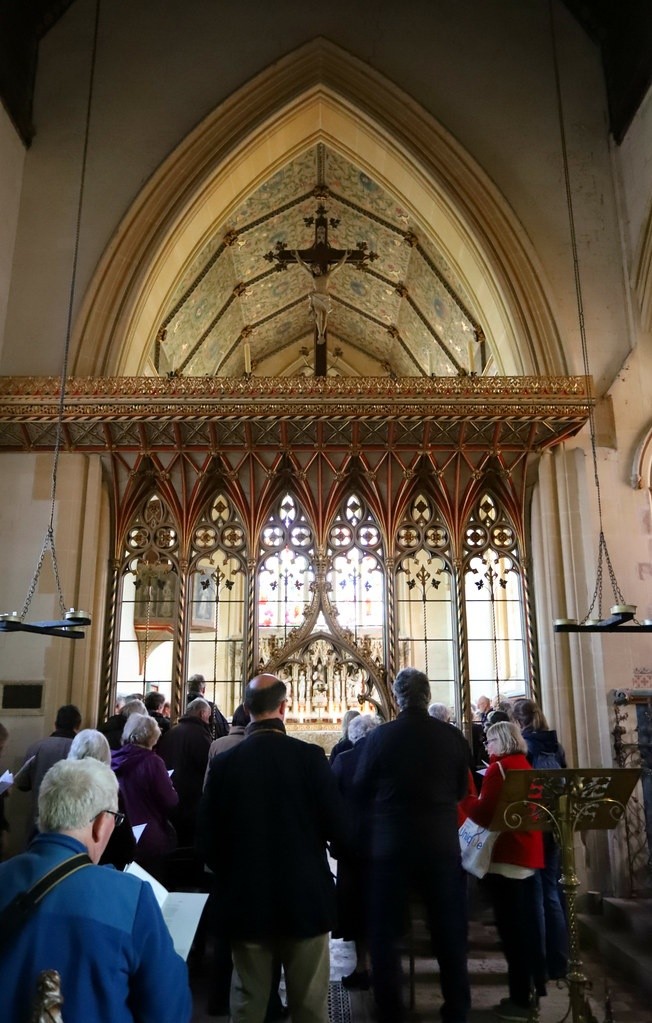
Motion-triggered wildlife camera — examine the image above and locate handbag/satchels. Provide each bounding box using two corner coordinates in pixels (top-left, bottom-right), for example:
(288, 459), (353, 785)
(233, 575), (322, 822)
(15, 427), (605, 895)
(458, 818), (500, 878)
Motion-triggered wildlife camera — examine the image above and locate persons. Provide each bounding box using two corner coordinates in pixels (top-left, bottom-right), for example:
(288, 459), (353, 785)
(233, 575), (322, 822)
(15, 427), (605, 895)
(0, 674), (288, 1023)
(200, 672), (336, 1023)
(0, 758), (195, 1023)
(328, 668), (569, 1020)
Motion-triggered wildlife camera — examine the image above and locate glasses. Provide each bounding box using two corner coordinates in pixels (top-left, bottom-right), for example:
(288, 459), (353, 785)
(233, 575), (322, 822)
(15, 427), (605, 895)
(482, 738), (499, 747)
(89, 810), (125, 825)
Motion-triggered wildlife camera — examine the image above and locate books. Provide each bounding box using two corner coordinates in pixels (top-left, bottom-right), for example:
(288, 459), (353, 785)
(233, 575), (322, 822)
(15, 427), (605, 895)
(117, 860), (210, 966)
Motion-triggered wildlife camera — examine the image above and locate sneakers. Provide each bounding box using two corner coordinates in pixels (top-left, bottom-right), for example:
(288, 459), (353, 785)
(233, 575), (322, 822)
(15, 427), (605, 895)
(495, 997), (540, 1022)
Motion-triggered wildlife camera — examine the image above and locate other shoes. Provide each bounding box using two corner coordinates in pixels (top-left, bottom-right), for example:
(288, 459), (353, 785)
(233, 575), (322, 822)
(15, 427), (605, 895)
(265, 1003), (291, 1023)
(340, 955), (402, 988)
(371, 998), (406, 1023)
(207, 1000), (231, 1016)
(441, 1002), (466, 1022)
